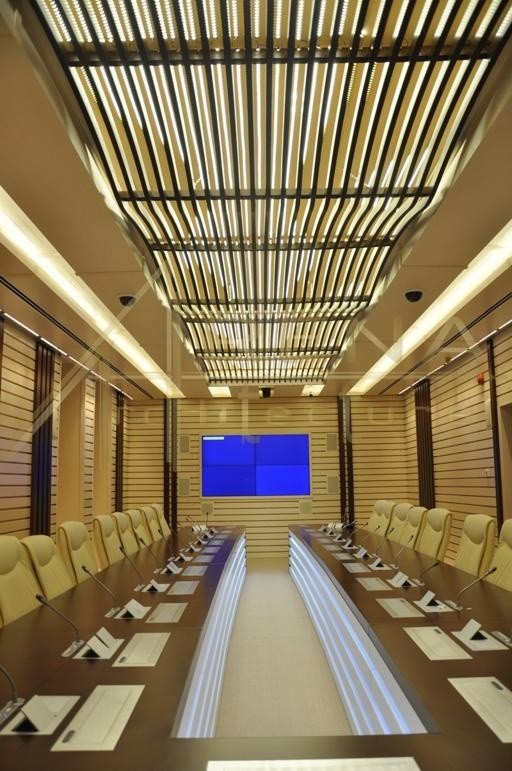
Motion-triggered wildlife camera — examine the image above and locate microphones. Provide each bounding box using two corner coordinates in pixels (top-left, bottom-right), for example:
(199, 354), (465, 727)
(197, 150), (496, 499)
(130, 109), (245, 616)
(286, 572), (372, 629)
(347, 522), (368, 541)
(120, 547), (145, 592)
(81, 564), (125, 619)
(158, 529), (175, 562)
(389, 535), (413, 570)
(35, 594), (84, 658)
(357, 525), (380, 548)
(140, 538), (161, 573)
(371, 528), (394, 558)
(343, 520), (357, 529)
(444, 566), (497, 612)
(412, 563), (439, 587)
(168, 515), (204, 553)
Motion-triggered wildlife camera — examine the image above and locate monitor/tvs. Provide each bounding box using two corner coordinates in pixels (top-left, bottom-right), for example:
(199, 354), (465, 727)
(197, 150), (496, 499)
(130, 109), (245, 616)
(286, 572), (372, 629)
(202, 435), (310, 495)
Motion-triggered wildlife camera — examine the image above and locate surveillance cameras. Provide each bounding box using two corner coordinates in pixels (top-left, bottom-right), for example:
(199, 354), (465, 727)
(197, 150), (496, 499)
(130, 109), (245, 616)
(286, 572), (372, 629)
(405, 289), (423, 305)
(117, 294), (138, 309)
